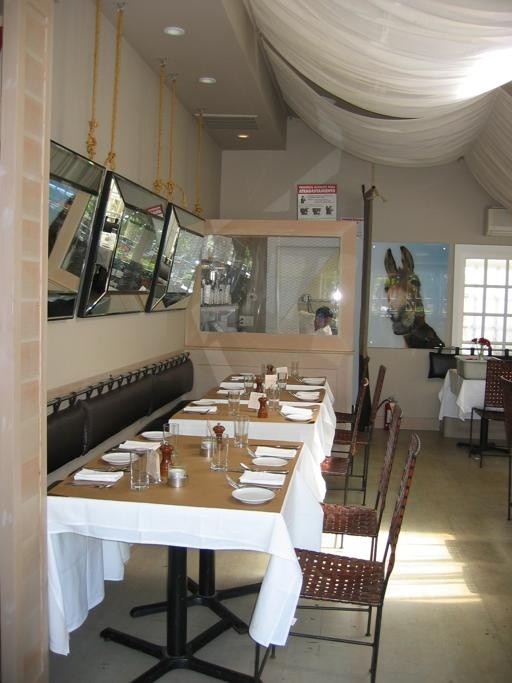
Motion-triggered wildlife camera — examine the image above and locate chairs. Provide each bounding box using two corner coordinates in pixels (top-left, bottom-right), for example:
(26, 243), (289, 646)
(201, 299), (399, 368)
(319, 404), (404, 561)
(468, 359), (511, 468)
(320, 377), (369, 548)
(255, 432), (421, 683)
(333, 365), (387, 505)
(335, 356), (371, 475)
(501, 375), (512, 521)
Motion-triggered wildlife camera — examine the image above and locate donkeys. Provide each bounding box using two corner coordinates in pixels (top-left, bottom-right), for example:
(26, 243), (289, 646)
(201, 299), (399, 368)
(384, 245), (445, 349)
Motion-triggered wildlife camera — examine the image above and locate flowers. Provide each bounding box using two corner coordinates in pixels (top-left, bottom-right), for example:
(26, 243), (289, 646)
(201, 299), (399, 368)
(472, 338), (492, 359)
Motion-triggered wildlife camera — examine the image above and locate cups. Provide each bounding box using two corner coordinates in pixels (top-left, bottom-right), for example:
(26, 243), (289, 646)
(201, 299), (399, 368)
(228, 374), (254, 448)
(130, 448), (187, 493)
(201, 431), (229, 473)
(269, 359), (299, 410)
(163, 423), (178, 443)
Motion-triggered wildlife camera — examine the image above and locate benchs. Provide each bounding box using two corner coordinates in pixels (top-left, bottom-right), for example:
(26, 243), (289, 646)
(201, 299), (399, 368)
(46, 351), (200, 495)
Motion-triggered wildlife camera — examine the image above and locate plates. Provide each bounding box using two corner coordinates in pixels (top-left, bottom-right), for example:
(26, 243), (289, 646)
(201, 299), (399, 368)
(101, 452), (131, 466)
(230, 457), (288, 504)
(286, 377), (325, 422)
(239, 373), (253, 375)
(191, 400), (216, 406)
(141, 431), (172, 441)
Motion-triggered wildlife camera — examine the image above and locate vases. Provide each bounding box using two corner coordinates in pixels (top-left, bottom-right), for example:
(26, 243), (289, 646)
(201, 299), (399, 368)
(454, 354), (504, 379)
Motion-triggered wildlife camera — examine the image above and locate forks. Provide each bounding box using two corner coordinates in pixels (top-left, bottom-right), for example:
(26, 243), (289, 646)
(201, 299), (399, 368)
(225, 474), (280, 489)
(182, 410), (210, 415)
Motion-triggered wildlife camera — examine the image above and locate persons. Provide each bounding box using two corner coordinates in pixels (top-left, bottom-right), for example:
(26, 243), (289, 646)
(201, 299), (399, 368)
(311, 307), (333, 335)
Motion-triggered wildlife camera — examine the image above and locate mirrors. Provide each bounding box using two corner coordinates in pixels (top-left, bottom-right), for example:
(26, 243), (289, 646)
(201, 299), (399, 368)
(77, 170), (169, 319)
(48, 140), (106, 321)
(146, 202), (204, 312)
(184, 220), (357, 353)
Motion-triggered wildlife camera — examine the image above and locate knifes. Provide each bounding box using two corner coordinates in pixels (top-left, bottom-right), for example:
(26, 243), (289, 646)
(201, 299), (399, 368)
(250, 443), (302, 449)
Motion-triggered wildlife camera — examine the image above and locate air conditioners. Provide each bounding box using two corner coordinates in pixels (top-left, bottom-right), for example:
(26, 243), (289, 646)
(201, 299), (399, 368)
(484, 207), (512, 239)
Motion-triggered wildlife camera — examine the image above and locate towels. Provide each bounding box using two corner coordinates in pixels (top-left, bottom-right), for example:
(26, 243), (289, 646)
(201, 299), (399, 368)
(73, 468), (123, 483)
(119, 439), (163, 450)
(184, 405), (218, 413)
(254, 445), (297, 459)
(238, 470), (287, 486)
